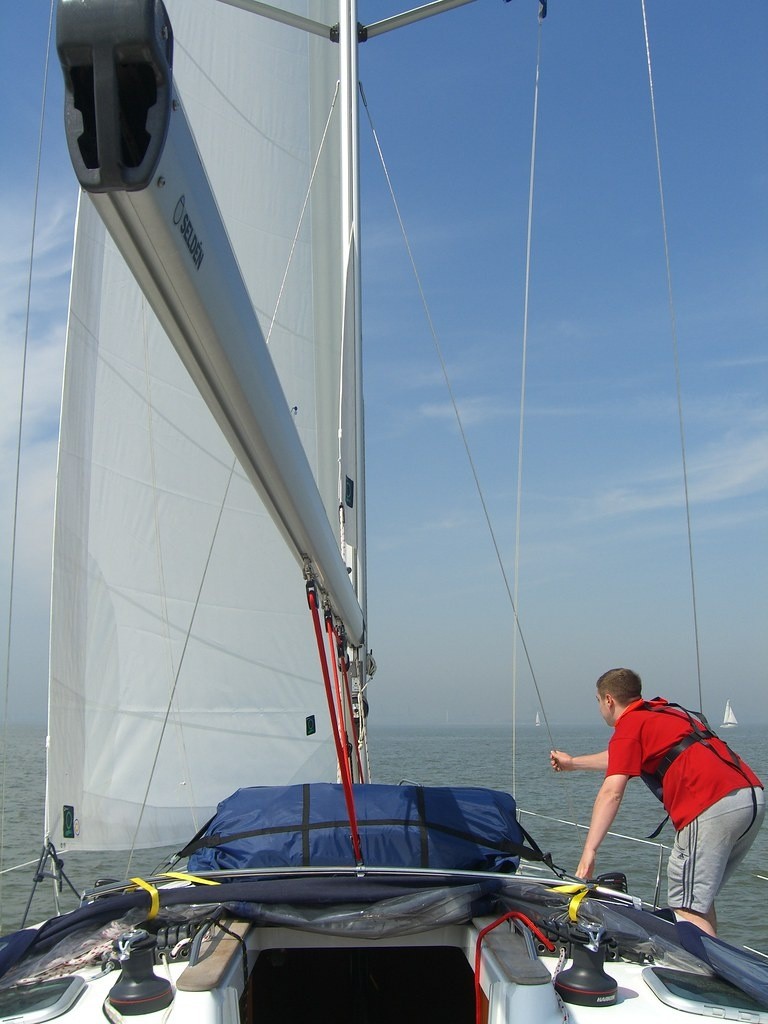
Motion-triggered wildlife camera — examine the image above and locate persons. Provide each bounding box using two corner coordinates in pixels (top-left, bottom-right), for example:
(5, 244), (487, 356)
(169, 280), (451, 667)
(549, 667), (767, 938)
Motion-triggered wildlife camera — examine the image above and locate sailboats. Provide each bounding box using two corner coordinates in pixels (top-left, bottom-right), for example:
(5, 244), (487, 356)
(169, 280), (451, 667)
(535, 711), (541, 727)
(719, 699), (738, 729)
